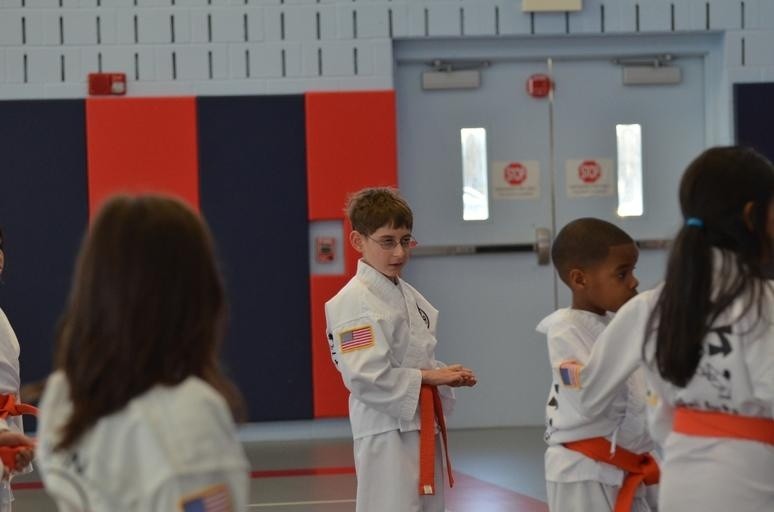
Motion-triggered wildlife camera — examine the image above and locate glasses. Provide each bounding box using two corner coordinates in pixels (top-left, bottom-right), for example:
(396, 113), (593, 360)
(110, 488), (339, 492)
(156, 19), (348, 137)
(368, 236), (418, 251)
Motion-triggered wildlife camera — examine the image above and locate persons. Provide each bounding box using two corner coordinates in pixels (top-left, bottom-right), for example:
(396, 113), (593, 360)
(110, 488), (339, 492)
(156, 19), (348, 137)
(34, 191), (257, 512)
(571, 142), (773, 512)
(534, 216), (660, 512)
(324, 184), (481, 511)
(0, 245), (37, 512)
